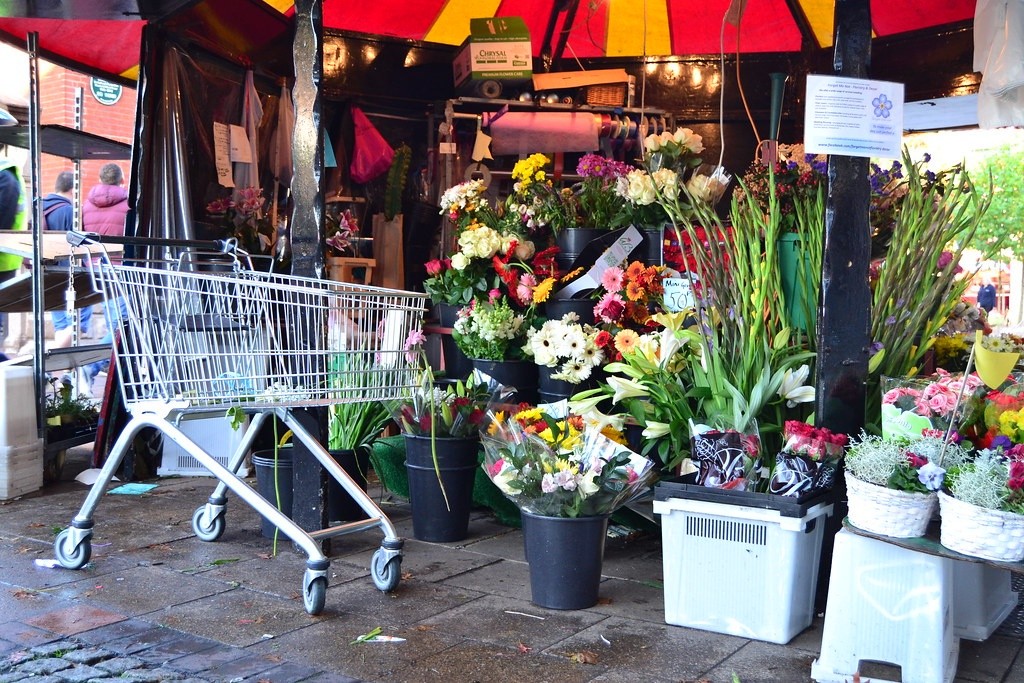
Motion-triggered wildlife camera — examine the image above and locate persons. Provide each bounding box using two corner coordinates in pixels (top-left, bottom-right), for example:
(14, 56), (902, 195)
(0, 156), (26, 363)
(82, 163), (130, 394)
(40, 171), (91, 391)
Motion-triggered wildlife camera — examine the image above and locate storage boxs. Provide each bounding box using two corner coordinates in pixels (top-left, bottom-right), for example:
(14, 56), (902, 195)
(453, 16), (532, 90)
(157, 414), (254, 479)
(652, 472), (834, 645)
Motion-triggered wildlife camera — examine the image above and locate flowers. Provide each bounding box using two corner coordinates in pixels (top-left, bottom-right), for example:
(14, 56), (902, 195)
(207, 186), (272, 252)
(257, 128), (1024, 557)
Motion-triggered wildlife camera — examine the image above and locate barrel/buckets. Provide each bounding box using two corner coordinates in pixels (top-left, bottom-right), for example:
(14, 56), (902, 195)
(250, 449), (296, 541)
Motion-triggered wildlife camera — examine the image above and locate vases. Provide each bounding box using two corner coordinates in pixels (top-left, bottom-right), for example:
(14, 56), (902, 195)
(328, 450), (369, 522)
(404, 460), (477, 542)
(521, 510), (613, 610)
(402, 433), (479, 468)
(844, 470), (937, 538)
(535, 364), (575, 394)
(438, 303), (462, 328)
(252, 449), (293, 541)
(778, 232), (817, 330)
(471, 358), (515, 385)
(637, 229), (664, 268)
(937, 490), (1024, 563)
(537, 389), (570, 404)
(546, 298), (597, 327)
(556, 228), (594, 270)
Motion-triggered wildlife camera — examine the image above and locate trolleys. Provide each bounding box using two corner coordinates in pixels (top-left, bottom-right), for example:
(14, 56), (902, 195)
(52, 230), (430, 616)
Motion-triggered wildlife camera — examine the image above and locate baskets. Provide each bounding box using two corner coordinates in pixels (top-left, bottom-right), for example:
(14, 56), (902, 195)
(844, 470), (936, 539)
(936, 489), (1024, 563)
(584, 82), (626, 106)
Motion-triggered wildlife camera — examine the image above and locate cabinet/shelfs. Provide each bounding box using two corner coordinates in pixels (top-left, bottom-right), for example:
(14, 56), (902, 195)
(0, 123), (133, 483)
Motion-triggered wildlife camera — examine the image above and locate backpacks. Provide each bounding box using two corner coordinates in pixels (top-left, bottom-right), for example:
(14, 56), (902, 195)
(22, 202), (67, 269)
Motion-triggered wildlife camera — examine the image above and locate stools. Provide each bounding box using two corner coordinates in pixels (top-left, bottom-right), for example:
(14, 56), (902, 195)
(810, 524), (1019, 683)
